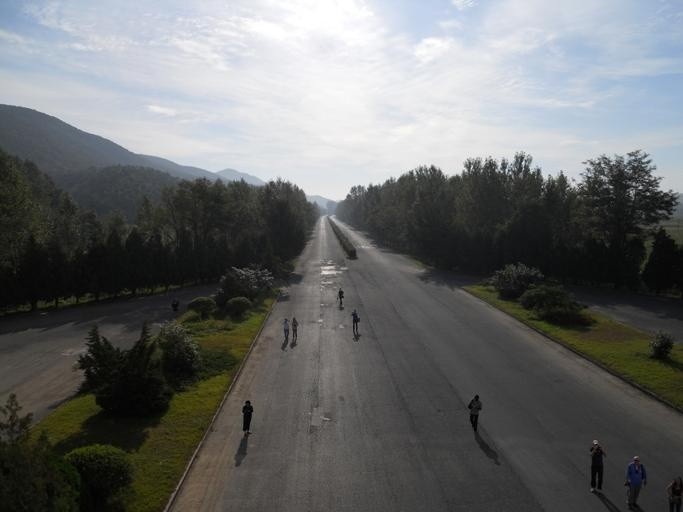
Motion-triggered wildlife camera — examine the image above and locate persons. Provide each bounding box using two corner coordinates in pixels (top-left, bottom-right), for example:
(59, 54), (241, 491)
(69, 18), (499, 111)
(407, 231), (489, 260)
(337, 287), (344, 308)
(352, 309), (359, 338)
(242, 400), (253, 437)
(290, 316), (299, 340)
(589, 440), (607, 493)
(468, 395), (482, 433)
(624, 455), (647, 510)
(283, 318), (290, 339)
(665, 477), (683, 512)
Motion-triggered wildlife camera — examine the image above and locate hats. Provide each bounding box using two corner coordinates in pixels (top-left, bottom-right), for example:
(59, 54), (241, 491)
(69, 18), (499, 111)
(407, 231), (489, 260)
(593, 440), (599, 445)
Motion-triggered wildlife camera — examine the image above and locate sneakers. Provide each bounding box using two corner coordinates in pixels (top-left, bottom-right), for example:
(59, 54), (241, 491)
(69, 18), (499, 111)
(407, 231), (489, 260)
(627, 504), (632, 509)
(591, 487), (594, 492)
(633, 502), (639, 508)
